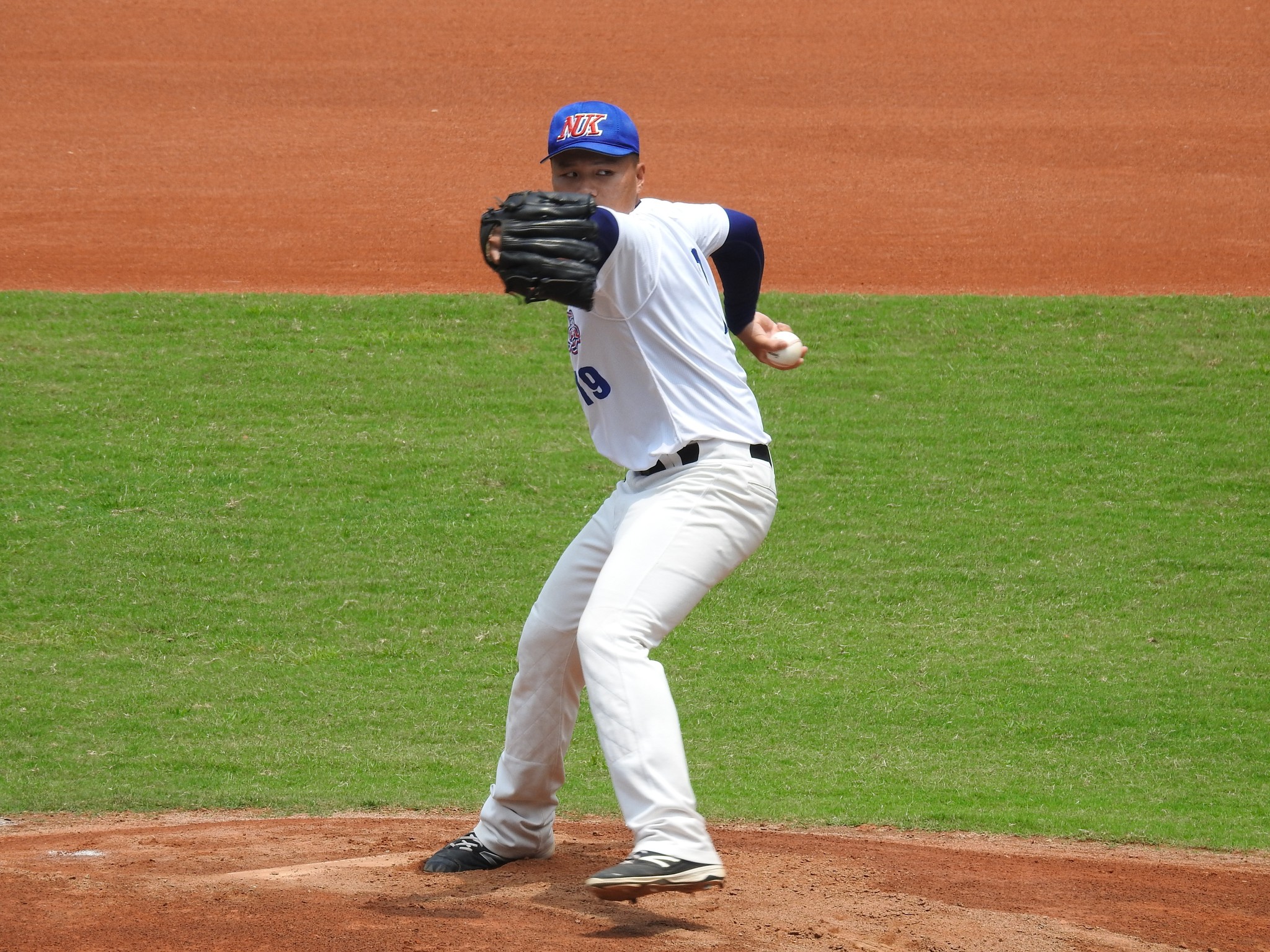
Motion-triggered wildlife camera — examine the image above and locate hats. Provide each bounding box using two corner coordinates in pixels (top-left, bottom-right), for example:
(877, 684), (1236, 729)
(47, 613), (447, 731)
(538, 99), (640, 163)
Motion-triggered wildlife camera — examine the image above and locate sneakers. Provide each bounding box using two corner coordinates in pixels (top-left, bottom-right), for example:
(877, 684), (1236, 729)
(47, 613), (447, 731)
(421, 831), (555, 871)
(586, 848), (727, 903)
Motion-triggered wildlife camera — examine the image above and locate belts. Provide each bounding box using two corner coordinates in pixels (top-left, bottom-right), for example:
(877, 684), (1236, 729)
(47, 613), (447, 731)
(636, 441), (772, 477)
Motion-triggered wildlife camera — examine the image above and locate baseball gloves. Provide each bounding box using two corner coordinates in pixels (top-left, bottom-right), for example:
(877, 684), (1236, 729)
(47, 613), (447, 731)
(477, 190), (601, 312)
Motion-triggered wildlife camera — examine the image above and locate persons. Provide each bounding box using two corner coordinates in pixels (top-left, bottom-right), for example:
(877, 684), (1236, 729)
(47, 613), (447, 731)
(421, 100), (809, 904)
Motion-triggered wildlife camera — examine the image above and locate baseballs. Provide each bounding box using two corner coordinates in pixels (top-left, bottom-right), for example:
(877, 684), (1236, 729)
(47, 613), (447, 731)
(767, 331), (803, 368)
